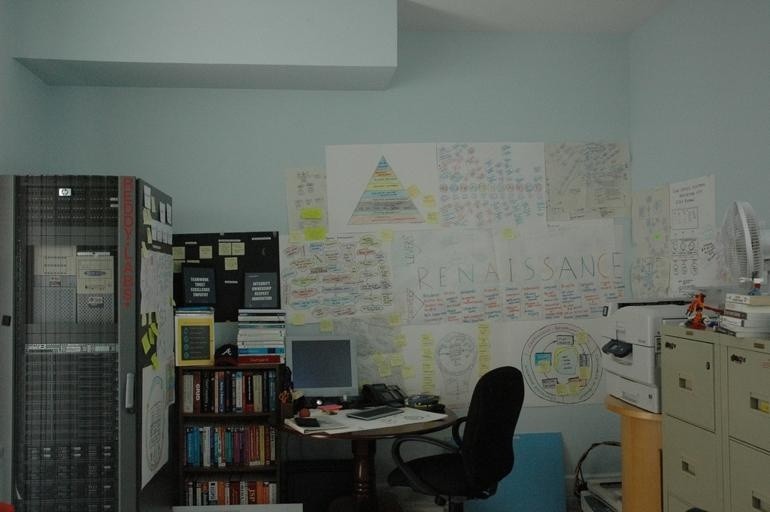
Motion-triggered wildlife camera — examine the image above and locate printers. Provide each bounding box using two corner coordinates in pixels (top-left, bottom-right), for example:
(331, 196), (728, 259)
(601, 296), (725, 414)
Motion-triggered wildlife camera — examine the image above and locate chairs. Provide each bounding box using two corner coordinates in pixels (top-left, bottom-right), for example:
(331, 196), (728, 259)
(383, 365), (526, 512)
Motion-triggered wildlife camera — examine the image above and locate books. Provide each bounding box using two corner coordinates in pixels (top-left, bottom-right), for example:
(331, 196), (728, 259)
(183, 308), (286, 506)
(713, 293), (770, 338)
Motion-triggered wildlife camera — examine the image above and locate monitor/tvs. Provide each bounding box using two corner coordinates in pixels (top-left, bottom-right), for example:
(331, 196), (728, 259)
(285, 335), (359, 409)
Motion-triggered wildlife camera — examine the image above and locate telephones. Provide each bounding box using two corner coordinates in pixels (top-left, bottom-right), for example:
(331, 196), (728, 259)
(362, 384), (395, 406)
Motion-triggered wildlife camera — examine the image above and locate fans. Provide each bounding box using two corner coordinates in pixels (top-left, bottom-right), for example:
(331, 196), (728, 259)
(719, 199), (770, 291)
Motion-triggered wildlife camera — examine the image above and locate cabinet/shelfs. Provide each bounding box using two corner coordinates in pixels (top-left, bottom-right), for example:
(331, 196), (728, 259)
(175, 364), (282, 507)
(660, 324), (770, 511)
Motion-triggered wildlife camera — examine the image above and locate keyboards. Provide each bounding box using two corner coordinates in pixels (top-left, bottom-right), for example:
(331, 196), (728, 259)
(346, 406), (404, 421)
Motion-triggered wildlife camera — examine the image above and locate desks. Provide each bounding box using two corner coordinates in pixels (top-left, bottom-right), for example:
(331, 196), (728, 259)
(604, 394), (663, 512)
(276, 405), (458, 511)
